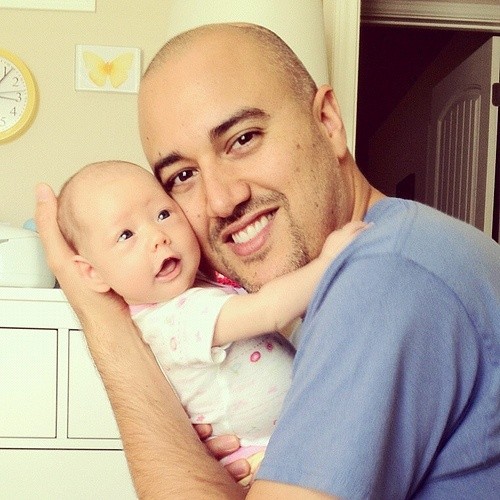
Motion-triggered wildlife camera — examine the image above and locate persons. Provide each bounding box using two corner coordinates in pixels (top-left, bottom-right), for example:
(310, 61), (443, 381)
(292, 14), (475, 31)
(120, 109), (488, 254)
(57, 161), (374, 489)
(36, 24), (500, 500)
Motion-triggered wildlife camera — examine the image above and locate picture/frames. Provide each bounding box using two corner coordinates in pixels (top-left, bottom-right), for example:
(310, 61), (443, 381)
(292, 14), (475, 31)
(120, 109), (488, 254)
(74, 43), (141, 94)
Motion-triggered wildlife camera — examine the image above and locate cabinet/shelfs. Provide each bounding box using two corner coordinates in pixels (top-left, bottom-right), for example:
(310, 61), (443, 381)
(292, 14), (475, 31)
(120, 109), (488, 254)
(2, 286), (126, 451)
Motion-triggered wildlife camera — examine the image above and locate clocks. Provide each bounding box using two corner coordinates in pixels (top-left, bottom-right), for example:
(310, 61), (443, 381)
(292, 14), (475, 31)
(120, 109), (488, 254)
(0, 49), (35, 140)
